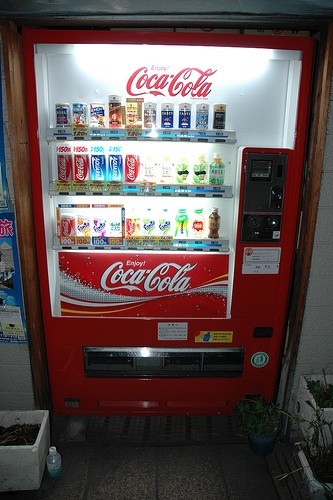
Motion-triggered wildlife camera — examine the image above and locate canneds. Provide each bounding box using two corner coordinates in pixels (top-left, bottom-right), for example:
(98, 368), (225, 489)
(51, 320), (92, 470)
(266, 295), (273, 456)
(61, 214), (121, 237)
(55, 102), (106, 127)
(57, 153), (141, 183)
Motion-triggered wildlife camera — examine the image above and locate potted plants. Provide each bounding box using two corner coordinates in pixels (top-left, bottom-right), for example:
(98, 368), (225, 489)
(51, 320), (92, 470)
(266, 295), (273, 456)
(272, 400), (333, 500)
(294, 368), (333, 456)
(235, 393), (280, 468)
(0, 410), (50, 491)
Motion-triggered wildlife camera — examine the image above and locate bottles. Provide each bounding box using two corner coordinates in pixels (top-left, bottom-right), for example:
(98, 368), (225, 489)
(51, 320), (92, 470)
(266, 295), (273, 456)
(176, 152), (225, 184)
(143, 207), (221, 238)
(46, 446), (62, 477)
